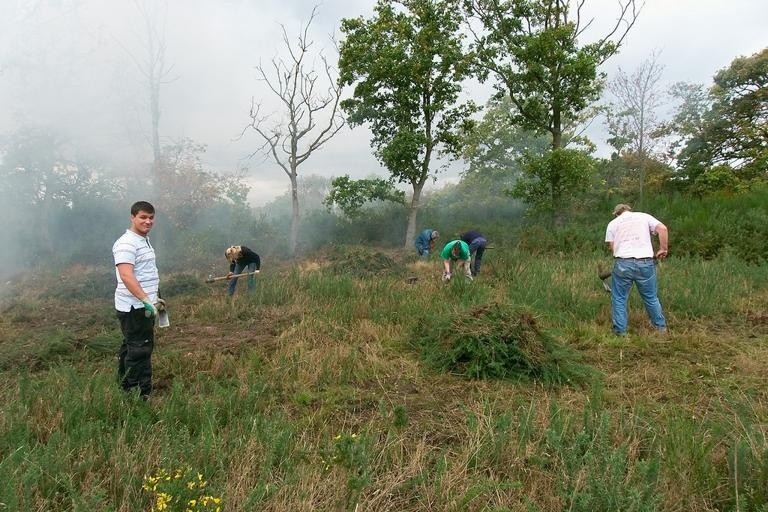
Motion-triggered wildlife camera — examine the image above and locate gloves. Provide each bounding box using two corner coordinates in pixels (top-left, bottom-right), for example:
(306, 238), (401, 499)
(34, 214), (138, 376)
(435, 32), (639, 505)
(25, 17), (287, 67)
(445, 273), (450, 280)
(158, 298), (165, 313)
(144, 296), (157, 320)
(226, 272), (233, 280)
(254, 269), (259, 275)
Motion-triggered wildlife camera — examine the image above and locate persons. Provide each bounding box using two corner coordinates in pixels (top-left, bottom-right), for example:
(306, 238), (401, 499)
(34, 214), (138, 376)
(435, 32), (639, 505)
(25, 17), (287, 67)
(224, 245), (260, 296)
(441, 240), (474, 283)
(460, 231), (486, 275)
(111, 201), (167, 401)
(606, 203), (670, 336)
(413, 229), (439, 259)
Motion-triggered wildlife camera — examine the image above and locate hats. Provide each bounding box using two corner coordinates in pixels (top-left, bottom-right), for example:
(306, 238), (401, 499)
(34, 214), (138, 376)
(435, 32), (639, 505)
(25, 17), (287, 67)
(612, 204), (631, 214)
